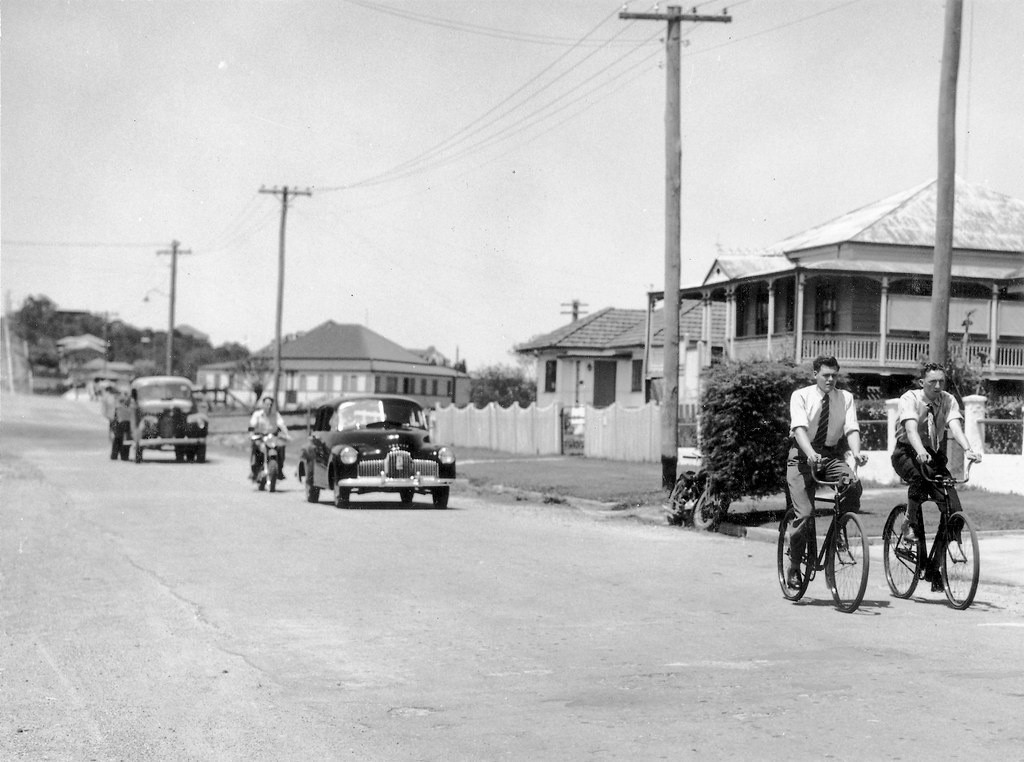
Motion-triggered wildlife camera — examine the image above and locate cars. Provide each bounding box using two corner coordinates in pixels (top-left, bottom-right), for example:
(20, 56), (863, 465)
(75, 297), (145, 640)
(294, 394), (456, 509)
(108, 376), (208, 464)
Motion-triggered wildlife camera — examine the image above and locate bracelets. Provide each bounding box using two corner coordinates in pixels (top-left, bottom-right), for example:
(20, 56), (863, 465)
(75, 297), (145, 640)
(965, 449), (973, 451)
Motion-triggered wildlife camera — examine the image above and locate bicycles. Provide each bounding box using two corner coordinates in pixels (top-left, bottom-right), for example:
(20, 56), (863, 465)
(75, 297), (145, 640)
(777, 456), (870, 614)
(881, 455), (980, 610)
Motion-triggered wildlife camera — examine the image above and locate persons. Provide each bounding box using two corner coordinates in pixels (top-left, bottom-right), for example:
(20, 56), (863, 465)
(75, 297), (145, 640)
(786, 355), (868, 590)
(890, 362), (983, 591)
(249, 397), (291, 480)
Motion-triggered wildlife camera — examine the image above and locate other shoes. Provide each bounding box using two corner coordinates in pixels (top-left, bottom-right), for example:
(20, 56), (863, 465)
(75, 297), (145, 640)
(901, 516), (919, 543)
(837, 535), (847, 549)
(924, 565), (946, 592)
(787, 566), (802, 589)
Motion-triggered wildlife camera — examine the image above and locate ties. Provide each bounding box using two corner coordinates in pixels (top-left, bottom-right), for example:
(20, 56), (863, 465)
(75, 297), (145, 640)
(811, 393), (830, 452)
(927, 404), (940, 453)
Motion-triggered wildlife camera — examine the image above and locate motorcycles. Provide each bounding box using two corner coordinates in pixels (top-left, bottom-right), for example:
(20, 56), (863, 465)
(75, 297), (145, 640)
(666, 470), (730, 531)
(247, 426), (286, 492)
